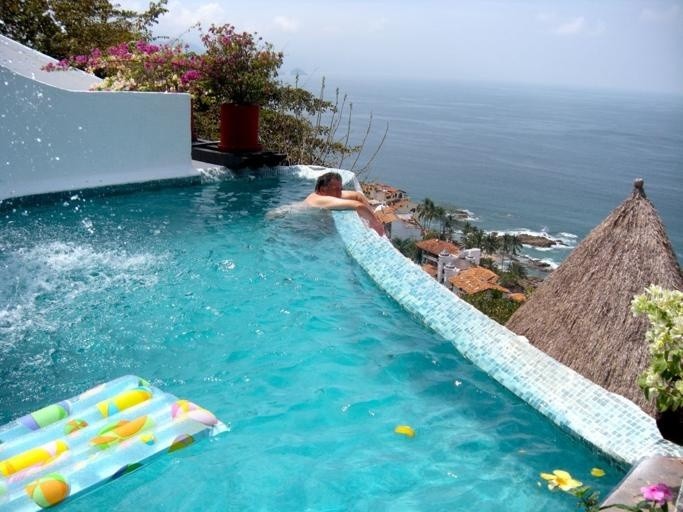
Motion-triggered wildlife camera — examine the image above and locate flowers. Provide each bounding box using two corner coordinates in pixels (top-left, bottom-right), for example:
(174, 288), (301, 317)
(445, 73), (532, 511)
(630, 285), (683, 412)
(201, 23), (283, 103)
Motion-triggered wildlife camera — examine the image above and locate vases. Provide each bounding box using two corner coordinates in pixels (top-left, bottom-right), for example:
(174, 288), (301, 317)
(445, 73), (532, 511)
(656, 406), (683, 449)
(218, 103), (261, 152)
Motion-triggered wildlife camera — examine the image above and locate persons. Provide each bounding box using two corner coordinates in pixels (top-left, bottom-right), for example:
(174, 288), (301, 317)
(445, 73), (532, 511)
(266, 172), (385, 237)
